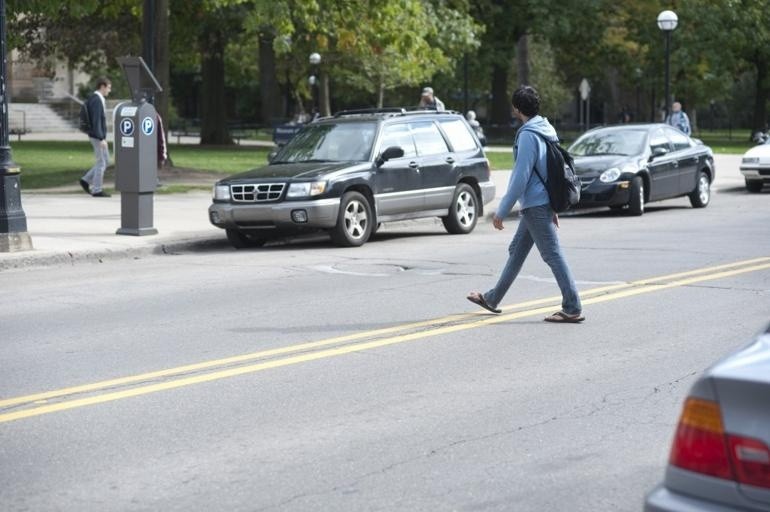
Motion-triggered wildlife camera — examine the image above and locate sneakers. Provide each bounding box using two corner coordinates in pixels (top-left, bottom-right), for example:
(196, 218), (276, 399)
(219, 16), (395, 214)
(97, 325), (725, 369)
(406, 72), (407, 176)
(93, 191), (111, 198)
(78, 178), (91, 195)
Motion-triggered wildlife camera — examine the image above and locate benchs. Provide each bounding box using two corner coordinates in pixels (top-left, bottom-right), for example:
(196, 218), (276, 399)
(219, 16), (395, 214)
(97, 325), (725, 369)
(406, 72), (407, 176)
(171, 129), (250, 146)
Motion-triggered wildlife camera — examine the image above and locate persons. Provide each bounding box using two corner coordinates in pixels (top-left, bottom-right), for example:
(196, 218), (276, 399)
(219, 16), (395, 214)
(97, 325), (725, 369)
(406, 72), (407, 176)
(665, 101), (692, 137)
(80, 77), (112, 197)
(157, 113), (168, 189)
(417, 86), (445, 110)
(466, 108), (486, 145)
(469, 84), (585, 323)
(753, 129), (768, 146)
(624, 114), (632, 124)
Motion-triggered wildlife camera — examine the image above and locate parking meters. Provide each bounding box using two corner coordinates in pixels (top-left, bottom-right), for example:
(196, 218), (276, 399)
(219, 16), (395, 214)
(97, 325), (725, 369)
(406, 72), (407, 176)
(107, 49), (171, 240)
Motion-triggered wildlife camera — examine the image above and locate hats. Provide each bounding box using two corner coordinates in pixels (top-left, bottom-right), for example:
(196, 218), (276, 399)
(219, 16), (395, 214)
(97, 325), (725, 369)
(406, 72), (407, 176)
(420, 87), (434, 97)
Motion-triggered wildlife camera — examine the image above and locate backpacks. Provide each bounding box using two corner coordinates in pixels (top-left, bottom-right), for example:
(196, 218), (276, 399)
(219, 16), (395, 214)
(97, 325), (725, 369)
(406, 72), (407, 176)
(526, 129), (582, 214)
(79, 94), (98, 134)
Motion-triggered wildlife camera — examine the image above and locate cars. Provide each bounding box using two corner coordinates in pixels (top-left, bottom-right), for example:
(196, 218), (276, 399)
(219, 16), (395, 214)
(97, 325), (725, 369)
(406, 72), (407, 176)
(559, 123), (718, 214)
(736, 132), (770, 196)
(642, 316), (768, 512)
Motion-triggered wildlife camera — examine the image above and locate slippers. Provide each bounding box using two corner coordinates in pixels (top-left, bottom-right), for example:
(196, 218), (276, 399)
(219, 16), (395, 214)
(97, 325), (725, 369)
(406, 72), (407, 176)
(466, 290), (503, 314)
(544, 309), (586, 323)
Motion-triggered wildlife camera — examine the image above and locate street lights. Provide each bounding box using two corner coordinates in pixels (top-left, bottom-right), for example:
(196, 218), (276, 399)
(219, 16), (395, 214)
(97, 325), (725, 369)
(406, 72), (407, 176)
(657, 9), (680, 129)
(307, 50), (325, 117)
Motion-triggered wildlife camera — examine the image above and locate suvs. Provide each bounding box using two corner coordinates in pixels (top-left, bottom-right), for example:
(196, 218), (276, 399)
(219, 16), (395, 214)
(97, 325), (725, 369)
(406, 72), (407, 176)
(208, 106), (498, 244)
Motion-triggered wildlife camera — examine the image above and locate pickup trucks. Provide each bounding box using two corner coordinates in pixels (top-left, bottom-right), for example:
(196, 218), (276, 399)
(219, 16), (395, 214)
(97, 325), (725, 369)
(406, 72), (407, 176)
(272, 120), (309, 145)
